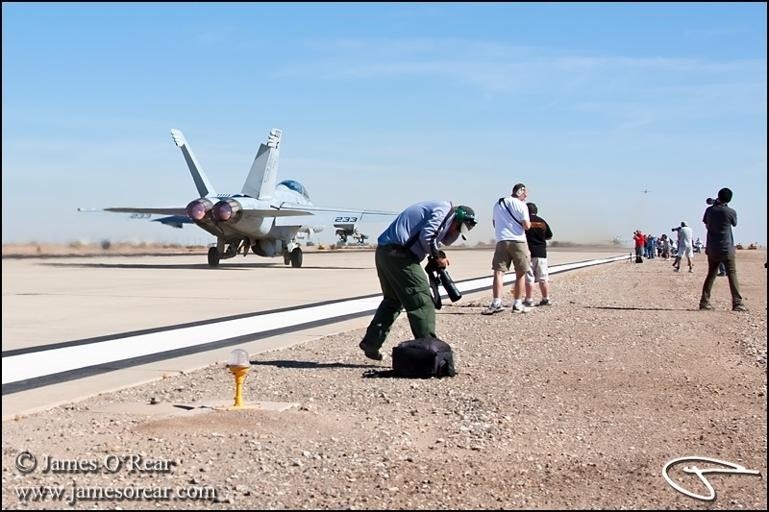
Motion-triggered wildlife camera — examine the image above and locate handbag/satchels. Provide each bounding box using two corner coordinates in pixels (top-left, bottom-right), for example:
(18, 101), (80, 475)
(636, 256), (643, 263)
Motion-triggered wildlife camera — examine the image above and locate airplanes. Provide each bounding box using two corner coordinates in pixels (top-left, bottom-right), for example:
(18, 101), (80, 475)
(68, 127), (407, 268)
(639, 189), (653, 194)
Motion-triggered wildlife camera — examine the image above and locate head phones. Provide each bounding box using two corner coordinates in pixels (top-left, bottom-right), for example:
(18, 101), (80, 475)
(516, 185), (525, 197)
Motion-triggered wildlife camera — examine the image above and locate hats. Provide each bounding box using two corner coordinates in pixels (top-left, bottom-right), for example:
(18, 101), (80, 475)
(457, 206), (477, 240)
(512, 183), (527, 197)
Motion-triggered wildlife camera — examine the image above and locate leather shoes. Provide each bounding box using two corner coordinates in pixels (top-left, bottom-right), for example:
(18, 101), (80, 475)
(359, 340), (383, 360)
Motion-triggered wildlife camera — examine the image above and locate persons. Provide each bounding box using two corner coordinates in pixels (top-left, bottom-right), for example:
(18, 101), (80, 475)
(482, 184), (533, 315)
(674, 221), (694, 273)
(522, 202), (553, 308)
(699, 187), (749, 312)
(359, 200), (477, 361)
(633, 229), (704, 263)
(717, 261), (728, 276)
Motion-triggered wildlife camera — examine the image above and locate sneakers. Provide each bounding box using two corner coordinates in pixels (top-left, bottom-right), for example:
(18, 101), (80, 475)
(673, 268), (680, 272)
(481, 296), (552, 316)
(700, 304), (712, 310)
(688, 269), (693, 273)
(717, 271), (726, 276)
(733, 304), (749, 310)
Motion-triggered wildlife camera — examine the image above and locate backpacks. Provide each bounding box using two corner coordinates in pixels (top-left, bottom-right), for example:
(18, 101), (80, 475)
(362, 333), (457, 379)
